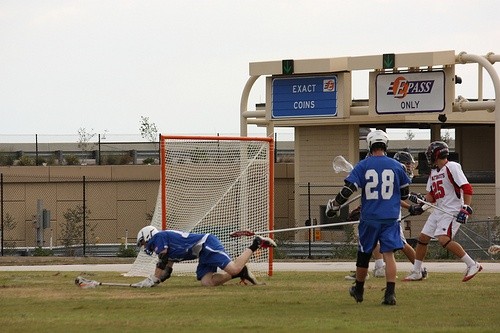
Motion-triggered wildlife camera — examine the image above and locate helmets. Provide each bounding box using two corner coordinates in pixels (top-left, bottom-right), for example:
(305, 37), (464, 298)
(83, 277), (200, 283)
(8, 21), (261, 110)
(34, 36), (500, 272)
(394, 151), (415, 164)
(426, 141), (449, 163)
(366, 130), (389, 151)
(137, 226), (159, 246)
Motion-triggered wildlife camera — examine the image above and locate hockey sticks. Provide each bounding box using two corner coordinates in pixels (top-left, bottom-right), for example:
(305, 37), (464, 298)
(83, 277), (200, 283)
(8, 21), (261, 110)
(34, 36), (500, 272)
(75, 277), (144, 288)
(227, 219), (361, 238)
(332, 156), (500, 265)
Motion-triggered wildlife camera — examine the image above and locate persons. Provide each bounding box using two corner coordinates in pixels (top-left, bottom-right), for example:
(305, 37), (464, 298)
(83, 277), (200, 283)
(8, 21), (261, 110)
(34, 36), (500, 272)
(326, 130), (483, 305)
(136, 225), (277, 289)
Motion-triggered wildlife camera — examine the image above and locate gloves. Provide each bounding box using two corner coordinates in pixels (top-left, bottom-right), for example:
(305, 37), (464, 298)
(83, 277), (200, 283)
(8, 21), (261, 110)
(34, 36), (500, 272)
(456, 205), (474, 224)
(409, 204), (424, 216)
(132, 274), (160, 288)
(409, 192), (426, 204)
(326, 199), (340, 218)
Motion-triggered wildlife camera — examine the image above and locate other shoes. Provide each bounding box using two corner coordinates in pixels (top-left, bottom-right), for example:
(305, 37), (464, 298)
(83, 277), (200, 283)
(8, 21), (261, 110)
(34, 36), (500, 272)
(254, 235), (277, 249)
(240, 264), (257, 285)
(375, 265), (385, 278)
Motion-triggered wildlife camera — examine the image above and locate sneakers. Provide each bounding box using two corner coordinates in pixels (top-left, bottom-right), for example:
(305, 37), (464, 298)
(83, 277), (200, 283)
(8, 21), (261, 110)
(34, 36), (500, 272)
(349, 286), (364, 303)
(462, 262), (483, 282)
(422, 267), (428, 279)
(381, 292), (396, 304)
(401, 271), (422, 281)
(345, 270), (370, 280)
